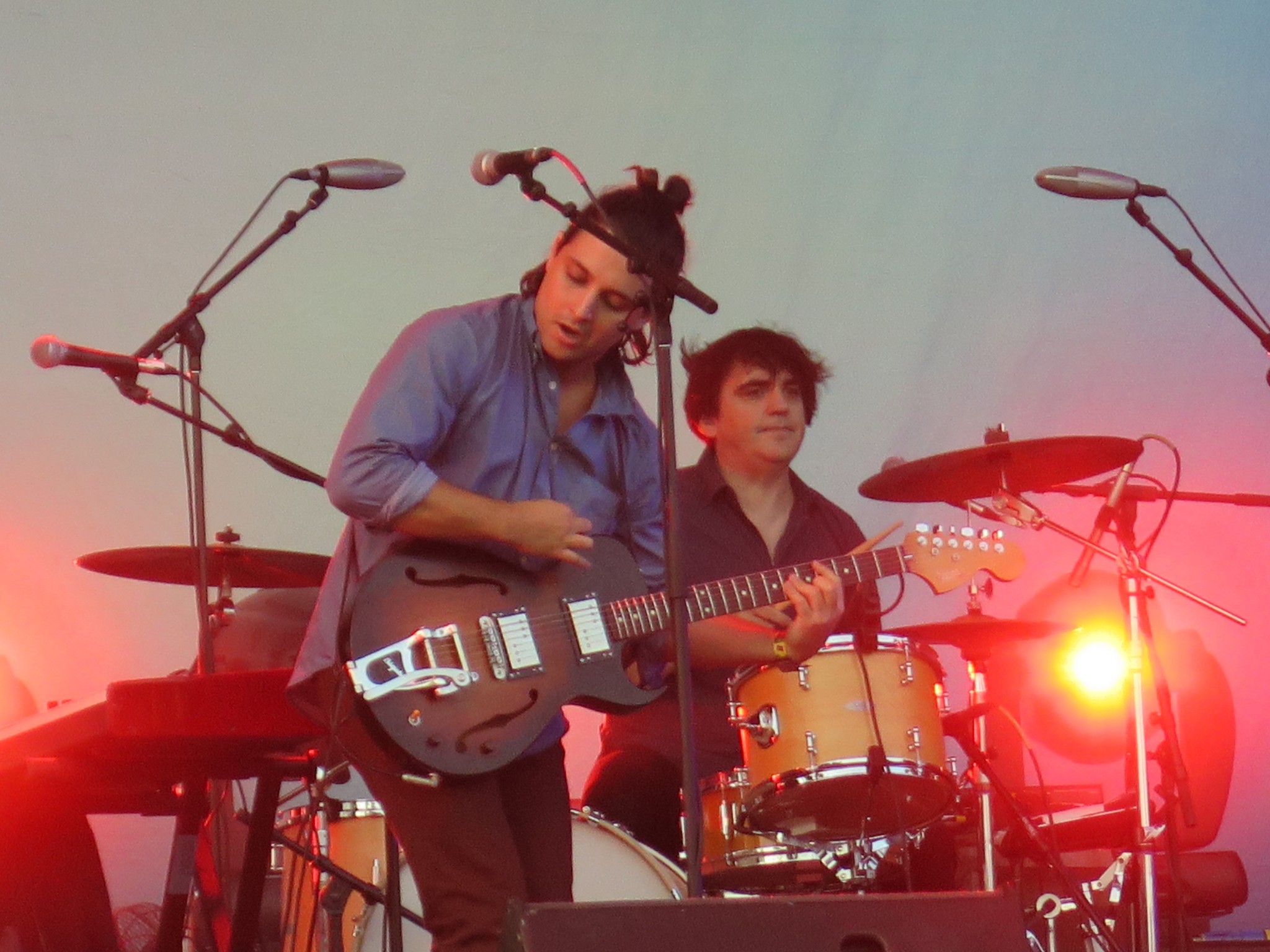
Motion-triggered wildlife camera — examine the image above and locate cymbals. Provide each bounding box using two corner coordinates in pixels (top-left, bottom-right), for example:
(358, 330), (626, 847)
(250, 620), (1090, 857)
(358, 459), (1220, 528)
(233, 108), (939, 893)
(884, 612), (1061, 645)
(76, 546), (331, 588)
(857, 434), (1142, 504)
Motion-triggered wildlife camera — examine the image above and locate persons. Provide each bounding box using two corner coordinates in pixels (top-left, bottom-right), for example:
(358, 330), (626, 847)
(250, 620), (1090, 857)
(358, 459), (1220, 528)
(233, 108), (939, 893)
(280, 182), (850, 951)
(576, 326), (885, 869)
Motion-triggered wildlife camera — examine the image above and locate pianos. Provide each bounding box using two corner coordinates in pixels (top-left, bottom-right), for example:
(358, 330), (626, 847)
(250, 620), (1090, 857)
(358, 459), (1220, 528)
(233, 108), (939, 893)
(0, 669), (333, 812)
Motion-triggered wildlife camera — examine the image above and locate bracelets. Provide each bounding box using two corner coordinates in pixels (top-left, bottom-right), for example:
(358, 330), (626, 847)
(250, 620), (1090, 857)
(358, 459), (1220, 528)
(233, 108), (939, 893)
(772, 631), (803, 674)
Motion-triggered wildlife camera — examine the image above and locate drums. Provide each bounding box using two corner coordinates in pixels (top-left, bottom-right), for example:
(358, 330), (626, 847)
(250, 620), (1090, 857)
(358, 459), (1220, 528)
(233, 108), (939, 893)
(724, 632), (962, 846)
(276, 799), (404, 951)
(350, 807), (688, 952)
(675, 765), (900, 883)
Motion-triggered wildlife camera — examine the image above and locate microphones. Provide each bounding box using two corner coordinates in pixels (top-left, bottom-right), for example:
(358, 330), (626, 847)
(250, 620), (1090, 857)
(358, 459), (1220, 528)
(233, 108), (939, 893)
(1069, 449), (1143, 589)
(1034, 167), (1168, 199)
(31, 335), (174, 375)
(470, 146), (551, 186)
(882, 455), (1000, 526)
(288, 158), (406, 189)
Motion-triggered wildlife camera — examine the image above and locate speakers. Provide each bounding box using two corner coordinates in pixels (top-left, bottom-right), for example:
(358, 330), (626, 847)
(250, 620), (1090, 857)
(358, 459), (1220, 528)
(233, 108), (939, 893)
(499, 887), (1031, 952)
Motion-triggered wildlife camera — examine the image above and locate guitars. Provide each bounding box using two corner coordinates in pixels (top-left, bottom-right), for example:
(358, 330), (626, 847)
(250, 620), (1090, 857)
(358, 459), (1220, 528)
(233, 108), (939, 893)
(339, 522), (1024, 779)
